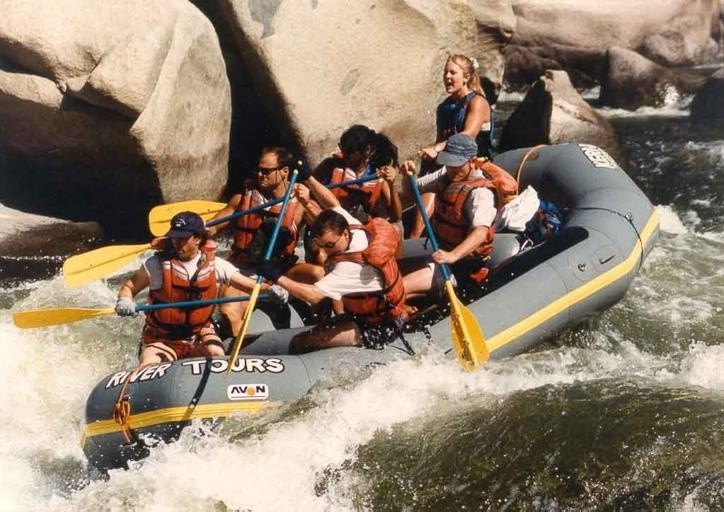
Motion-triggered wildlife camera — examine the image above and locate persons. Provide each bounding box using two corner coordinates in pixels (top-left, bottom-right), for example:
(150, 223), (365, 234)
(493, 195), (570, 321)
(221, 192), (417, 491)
(415, 53), (493, 193)
(115, 124), (517, 368)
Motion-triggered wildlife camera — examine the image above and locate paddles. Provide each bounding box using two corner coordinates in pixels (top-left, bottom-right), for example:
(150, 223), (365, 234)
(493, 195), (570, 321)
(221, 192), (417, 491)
(12, 293), (278, 329)
(225, 162), (299, 375)
(63, 193), (303, 289)
(149, 175), (384, 237)
(409, 168), (490, 373)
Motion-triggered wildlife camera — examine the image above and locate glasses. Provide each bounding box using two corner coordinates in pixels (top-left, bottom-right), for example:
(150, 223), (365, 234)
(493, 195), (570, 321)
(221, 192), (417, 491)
(314, 233), (344, 249)
(256, 168), (282, 175)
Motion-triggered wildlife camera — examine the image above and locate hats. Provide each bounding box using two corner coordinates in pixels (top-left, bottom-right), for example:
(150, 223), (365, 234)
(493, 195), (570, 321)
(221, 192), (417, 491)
(435, 134), (478, 167)
(163, 212), (204, 238)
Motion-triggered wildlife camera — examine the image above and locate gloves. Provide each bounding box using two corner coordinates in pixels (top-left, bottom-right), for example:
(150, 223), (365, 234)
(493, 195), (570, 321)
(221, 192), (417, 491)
(115, 298), (140, 317)
(268, 284), (290, 305)
(288, 158), (311, 180)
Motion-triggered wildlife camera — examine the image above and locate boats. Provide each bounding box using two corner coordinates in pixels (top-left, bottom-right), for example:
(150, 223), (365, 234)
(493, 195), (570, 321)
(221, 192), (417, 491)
(80, 141), (662, 477)
